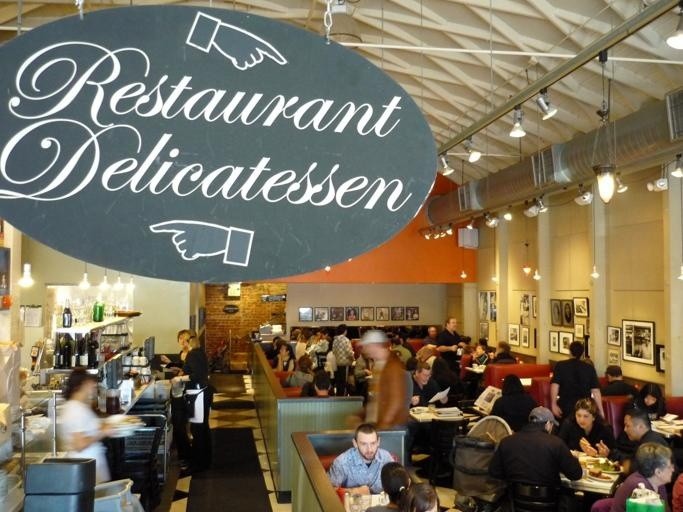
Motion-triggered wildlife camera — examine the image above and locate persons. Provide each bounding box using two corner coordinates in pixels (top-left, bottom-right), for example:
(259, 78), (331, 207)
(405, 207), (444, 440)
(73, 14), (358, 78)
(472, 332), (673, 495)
(550, 341), (605, 428)
(160, 330), (192, 467)
(171, 328), (215, 473)
(269, 314), (516, 408)
(552, 303), (560, 323)
(54, 368), (117, 488)
(359, 329), (410, 470)
(564, 303), (572, 323)
(347, 308), (356, 321)
(329, 424), (440, 512)
(490, 357), (683, 512)
(148, 220), (257, 266)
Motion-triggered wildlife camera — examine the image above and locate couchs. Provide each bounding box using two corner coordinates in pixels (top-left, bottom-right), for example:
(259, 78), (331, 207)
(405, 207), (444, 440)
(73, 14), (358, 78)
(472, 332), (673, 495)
(482, 363), (683, 441)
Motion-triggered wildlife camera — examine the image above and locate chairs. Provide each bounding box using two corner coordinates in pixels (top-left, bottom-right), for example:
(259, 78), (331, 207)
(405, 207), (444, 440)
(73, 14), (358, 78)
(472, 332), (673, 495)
(260, 339), (424, 501)
(508, 477), (560, 512)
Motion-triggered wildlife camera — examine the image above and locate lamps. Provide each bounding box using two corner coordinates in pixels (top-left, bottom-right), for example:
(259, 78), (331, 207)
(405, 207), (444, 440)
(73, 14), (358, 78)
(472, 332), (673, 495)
(18, 235), (124, 292)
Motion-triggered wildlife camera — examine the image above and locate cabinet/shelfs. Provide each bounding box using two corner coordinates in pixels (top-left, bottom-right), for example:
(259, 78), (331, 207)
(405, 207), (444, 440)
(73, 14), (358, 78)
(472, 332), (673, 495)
(0, 316), (155, 512)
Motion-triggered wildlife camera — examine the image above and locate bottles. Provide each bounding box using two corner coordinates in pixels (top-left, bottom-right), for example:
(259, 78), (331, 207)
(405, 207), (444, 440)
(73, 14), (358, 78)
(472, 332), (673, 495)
(56, 331), (98, 368)
(335, 482), (387, 512)
(72, 297), (128, 323)
(56, 297), (62, 328)
(62, 299), (72, 328)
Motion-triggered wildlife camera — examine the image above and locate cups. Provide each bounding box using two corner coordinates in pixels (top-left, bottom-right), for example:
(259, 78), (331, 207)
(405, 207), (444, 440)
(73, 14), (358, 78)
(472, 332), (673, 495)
(131, 356), (139, 366)
(105, 388), (121, 413)
(139, 357), (148, 366)
(123, 356), (131, 364)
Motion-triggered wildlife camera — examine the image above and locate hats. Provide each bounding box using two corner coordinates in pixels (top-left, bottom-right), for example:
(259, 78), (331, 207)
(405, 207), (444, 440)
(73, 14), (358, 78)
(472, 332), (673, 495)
(528, 405), (560, 426)
(359, 329), (385, 345)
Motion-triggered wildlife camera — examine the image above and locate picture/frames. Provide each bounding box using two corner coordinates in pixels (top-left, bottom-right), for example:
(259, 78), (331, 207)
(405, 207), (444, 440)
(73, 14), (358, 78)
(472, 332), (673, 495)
(299, 307), (419, 321)
(478, 291), (665, 373)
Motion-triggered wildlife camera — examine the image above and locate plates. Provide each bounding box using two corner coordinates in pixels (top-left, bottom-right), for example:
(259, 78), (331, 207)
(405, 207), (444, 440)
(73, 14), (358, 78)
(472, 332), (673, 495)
(585, 473), (612, 483)
(595, 462), (622, 474)
(649, 417), (682, 432)
